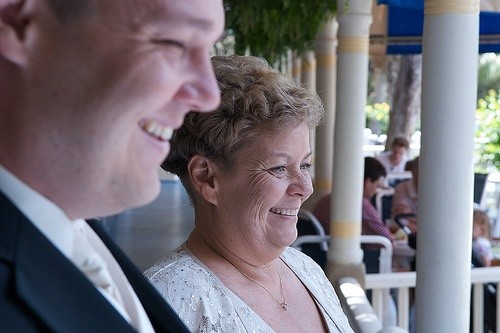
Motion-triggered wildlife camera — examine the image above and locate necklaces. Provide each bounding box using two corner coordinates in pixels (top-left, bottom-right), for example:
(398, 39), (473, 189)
(194, 228), (287, 309)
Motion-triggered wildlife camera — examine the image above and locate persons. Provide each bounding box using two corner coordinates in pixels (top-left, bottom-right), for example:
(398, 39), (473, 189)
(312, 158), (396, 274)
(392, 157), (416, 271)
(142, 54), (355, 333)
(472, 210), (493, 268)
(0, 0), (225, 333)
(373, 133), (416, 225)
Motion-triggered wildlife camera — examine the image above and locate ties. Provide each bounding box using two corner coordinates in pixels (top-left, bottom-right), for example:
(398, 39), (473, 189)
(60, 228), (132, 322)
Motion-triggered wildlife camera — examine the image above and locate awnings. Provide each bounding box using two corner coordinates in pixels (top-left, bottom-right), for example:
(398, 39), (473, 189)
(378, 0), (500, 56)
